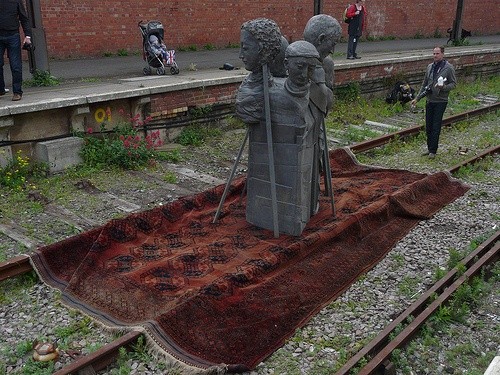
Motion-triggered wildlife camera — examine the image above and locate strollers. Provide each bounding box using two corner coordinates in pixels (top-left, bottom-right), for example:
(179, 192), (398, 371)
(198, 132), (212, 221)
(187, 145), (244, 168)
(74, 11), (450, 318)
(138, 19), (180, 76)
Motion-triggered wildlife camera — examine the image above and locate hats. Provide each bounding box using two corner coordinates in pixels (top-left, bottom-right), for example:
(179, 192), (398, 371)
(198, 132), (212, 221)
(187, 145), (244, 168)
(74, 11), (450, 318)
(150, 35), (158, 43)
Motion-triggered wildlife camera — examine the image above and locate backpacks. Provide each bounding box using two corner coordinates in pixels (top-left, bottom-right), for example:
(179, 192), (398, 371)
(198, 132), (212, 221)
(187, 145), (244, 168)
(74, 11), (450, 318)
(343, 4), (357, 24)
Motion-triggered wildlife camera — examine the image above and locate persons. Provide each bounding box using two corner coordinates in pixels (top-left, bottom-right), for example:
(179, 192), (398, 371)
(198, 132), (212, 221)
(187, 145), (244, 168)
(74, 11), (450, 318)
(321, 54), (335, 91)
(150, 35), (168, 58)
(346, 0), (367, 59)
(409, 45), (458, 159)
(233, 18), (282, 123)
(266, 40), (320, 127)
(0, 0), (32, 101)
(305, 13), (342, 116)
(267, 36), (290, 78)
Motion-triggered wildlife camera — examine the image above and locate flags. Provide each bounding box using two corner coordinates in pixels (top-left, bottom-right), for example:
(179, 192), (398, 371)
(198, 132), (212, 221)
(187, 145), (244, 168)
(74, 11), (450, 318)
(165, 49), (176, 64)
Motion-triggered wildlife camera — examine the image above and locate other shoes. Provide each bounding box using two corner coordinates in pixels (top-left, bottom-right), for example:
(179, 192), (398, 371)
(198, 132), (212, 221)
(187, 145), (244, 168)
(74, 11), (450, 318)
(421, 150), (430, 155)
(353, 56), (361, 59)
(0, 92), (5, 96)
(12, 94), (21, 101)
(347, 56), (355, 59)
(428, 152), (435, 159)
